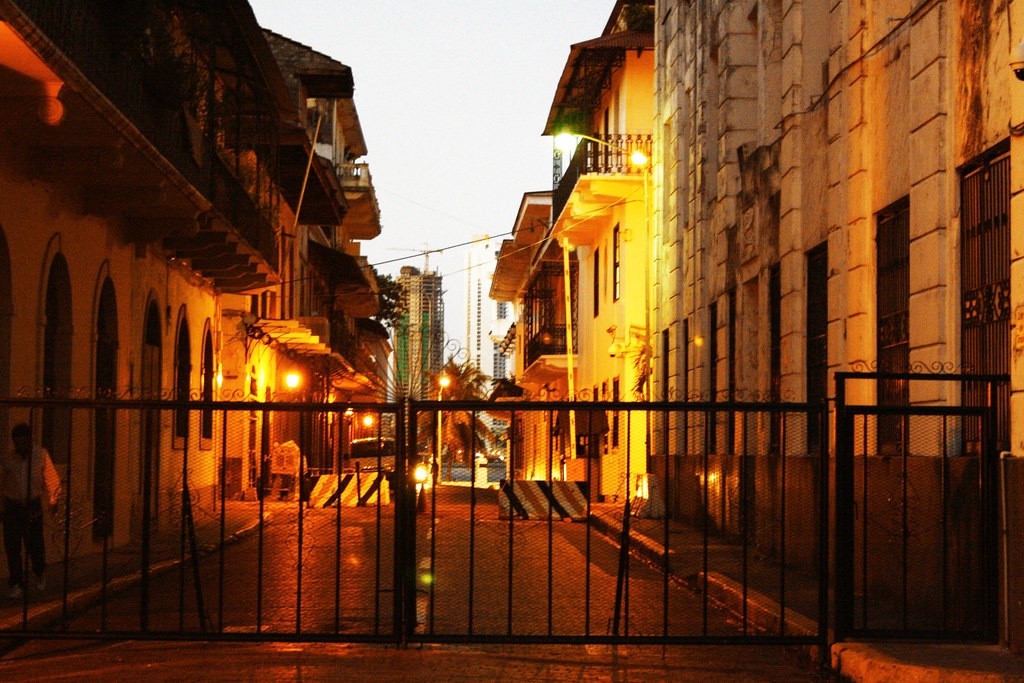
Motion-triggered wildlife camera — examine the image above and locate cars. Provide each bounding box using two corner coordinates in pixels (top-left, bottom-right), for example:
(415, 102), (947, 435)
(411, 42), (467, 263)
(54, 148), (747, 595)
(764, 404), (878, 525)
(345, 438), (397, 490)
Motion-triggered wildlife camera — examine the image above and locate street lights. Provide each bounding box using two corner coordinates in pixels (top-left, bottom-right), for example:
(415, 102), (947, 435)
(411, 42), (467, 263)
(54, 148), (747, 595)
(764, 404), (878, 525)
(435, 373), (451, 484)
